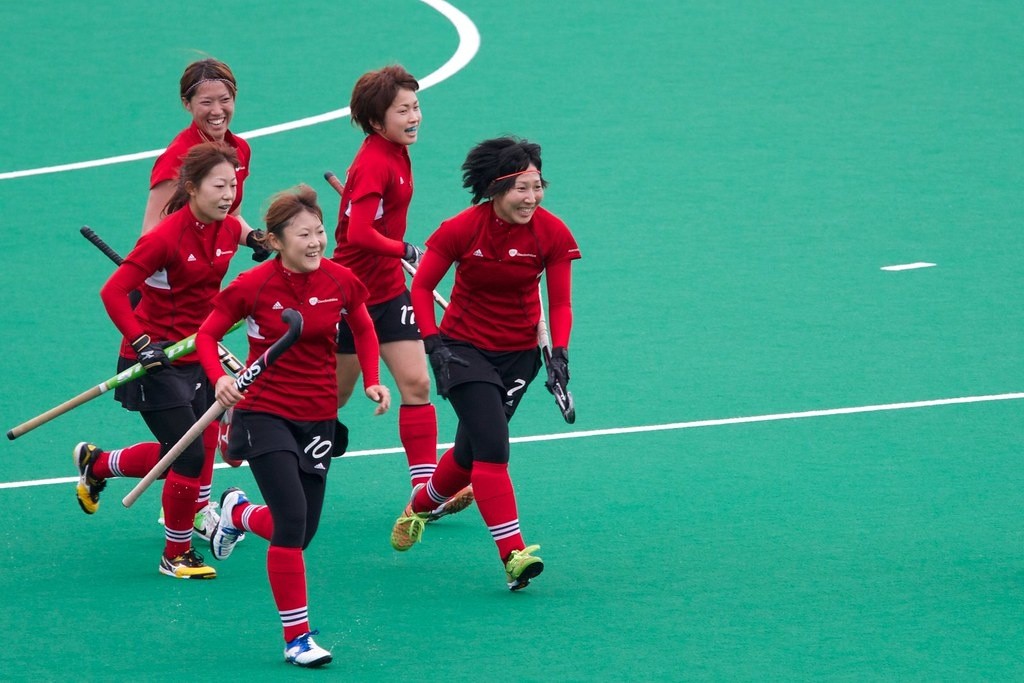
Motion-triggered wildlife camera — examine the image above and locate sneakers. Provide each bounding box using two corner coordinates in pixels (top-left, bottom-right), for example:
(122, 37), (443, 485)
(157, 501), (246, 543)
(430, 484), (474, 521)
(502, 543), (545, 593)
(208, 487), (250, 561)
(158, 549), (216, 580)
(282, 630), (333, 668)
(390, 481), (429, 553)
(73, 440), (106, 516)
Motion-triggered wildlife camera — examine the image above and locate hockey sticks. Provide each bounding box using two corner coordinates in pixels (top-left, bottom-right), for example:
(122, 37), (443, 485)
(537, 280), (577, 426)
(119, 306), (303, 509)
(79, 223), (248, 378)
(323, 170), (449, 310)
(5, 319), (246, 442)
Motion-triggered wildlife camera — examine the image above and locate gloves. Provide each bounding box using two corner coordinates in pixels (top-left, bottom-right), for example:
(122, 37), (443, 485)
(246, 228), (273, 263)
(427, 344), (471, 400)
(132, 332), (179, 376)
(546, 346), (576, 394)
(404, 241), (426, 268)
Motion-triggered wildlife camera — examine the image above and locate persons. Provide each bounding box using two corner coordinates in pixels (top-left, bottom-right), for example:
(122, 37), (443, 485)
(143, 60), (272, 546)
(75, 140), (239, 583)
(193, 185), (392, 671)
(220, 63), (472, 521)
(391, 135), (584, 592)
